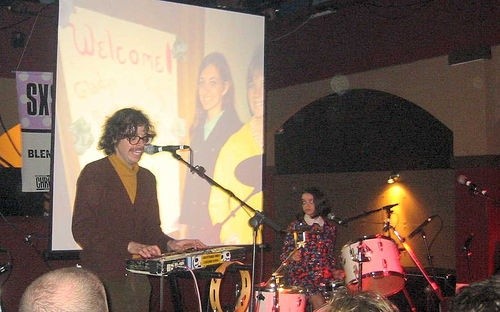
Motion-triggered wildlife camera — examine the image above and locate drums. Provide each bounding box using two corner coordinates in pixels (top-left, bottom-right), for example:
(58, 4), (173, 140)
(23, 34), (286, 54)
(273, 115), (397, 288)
(339, 233), (408, 298)
(254, 285), (309, 312)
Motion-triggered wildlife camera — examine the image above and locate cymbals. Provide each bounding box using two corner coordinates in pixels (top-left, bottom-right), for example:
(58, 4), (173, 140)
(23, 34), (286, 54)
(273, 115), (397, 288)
(280, 226), (326, 234)
(209, 260), (252, 312)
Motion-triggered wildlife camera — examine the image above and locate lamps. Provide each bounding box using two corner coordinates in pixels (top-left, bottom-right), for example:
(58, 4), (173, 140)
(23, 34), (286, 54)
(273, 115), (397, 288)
(388, 174), (400, 183)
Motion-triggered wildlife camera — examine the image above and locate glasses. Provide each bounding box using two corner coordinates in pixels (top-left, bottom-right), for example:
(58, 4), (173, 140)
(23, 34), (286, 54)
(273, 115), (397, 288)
(120, 133), (153, 145)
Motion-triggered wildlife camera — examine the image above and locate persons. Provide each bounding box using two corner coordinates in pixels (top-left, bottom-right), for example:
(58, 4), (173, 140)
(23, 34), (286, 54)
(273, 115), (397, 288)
(178, 52), (264, 244)
(447, 277), (500, 312)
(315, 293), (398, 312)
(71, 107), (208, 312)
(282, 187), (339, 309)
(19, 269), (110, 312)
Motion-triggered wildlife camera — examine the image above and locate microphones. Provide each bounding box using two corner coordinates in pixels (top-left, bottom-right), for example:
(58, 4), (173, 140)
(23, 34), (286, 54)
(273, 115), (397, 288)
(457, 175), (489, 196)
(463, 233), (474, 251)
(409, 216), (435, 239)
(145, 145), (189, 155)
(328, 213), (346, 227)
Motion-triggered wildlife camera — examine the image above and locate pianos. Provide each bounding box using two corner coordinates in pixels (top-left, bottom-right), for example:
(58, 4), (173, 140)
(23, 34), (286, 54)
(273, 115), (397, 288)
(125, 246), (248, 278)
